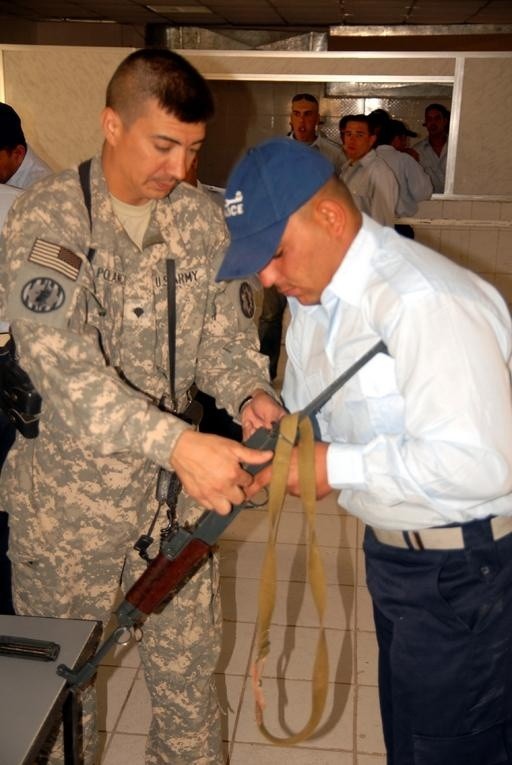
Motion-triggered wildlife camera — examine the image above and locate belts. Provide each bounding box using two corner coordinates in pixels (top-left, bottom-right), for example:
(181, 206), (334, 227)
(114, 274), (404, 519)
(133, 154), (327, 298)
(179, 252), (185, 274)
(371, 515), (512, 550)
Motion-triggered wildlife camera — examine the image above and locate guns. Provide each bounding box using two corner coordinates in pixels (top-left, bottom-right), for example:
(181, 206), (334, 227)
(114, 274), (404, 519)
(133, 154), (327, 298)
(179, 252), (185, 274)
(57, 339), (392, 699)
(0, 336), (42, 472)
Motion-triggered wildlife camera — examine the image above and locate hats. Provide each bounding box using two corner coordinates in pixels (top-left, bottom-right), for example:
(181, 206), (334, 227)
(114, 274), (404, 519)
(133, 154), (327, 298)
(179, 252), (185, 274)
(213, 138), (335, 281)
(371, 110), (417, 137)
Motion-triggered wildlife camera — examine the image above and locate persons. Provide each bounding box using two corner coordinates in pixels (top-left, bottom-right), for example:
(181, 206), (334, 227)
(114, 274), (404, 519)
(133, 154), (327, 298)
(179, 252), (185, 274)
(0, 43), (290, 762)
(414, 104), (450, 194)
(1, 103), (54, 194)
(215, 138), (512, 764)
(376, 121), (433, 241)
(336, 114), (398, 232)
(369, 109), (391, 149)
(287, 91), (348, 175)
